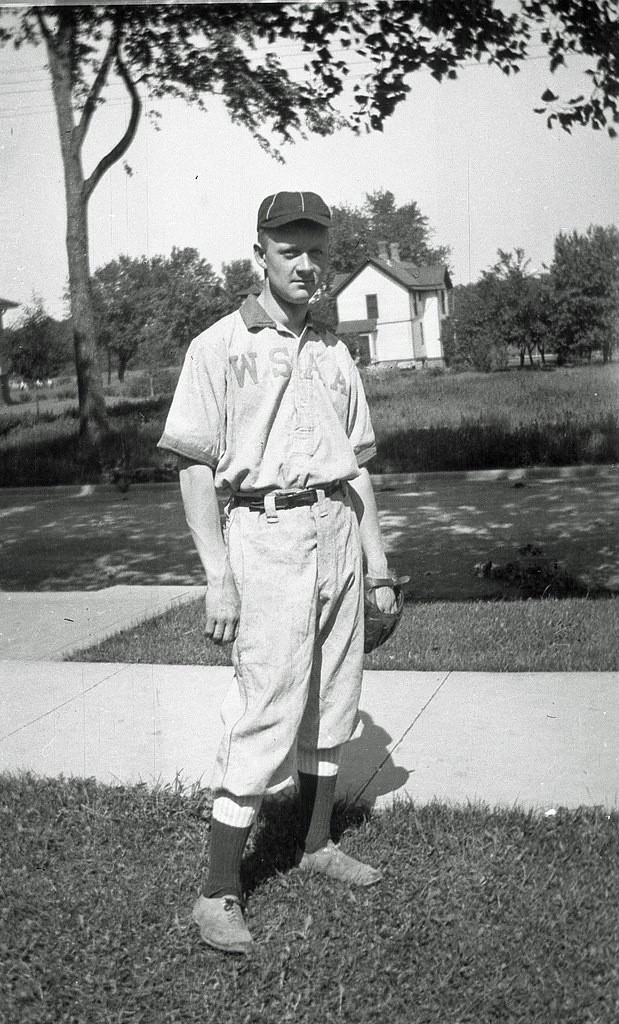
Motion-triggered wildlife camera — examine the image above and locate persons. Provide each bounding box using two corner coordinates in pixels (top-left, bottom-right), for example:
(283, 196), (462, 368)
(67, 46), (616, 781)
(177, 190), (399, 956)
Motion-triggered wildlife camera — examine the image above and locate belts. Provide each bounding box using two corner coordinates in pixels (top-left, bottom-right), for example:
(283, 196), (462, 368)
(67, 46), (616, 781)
(234, 484), (340, 511)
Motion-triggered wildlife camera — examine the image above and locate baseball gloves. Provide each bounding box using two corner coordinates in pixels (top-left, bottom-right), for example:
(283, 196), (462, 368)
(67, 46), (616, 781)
(362, 566), (412, 655)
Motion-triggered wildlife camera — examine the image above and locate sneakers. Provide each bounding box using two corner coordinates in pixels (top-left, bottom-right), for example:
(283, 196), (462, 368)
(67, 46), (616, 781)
(190, 892), (253, 952)
(296, 839), (384, 887)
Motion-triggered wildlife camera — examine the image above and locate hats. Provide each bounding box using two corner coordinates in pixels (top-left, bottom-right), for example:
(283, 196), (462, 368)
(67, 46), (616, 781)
(256, 191), (332, 231)
